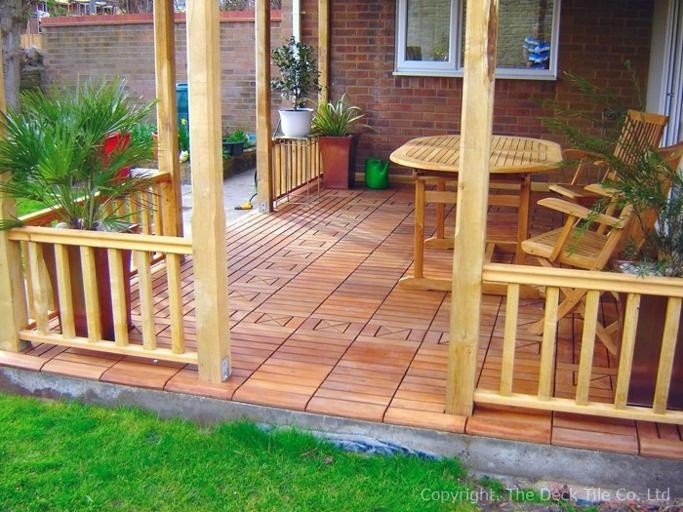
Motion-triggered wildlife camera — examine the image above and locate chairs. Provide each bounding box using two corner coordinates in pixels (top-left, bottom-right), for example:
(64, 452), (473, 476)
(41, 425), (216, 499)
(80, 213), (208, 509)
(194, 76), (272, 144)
(519, 141), (683, 377)
(549, 109), (669, 235)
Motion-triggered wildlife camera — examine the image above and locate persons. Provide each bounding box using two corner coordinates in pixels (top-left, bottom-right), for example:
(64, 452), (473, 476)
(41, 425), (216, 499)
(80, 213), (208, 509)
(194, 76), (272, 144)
(523, 22), (550, 69)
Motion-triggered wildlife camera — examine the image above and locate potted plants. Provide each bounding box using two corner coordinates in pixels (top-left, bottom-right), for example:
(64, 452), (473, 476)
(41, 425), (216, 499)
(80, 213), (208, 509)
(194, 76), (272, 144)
(0, 72), (165, 340)
(309, 93), (379, 190)
(270, 34), (324, 138)
(223, 128), (248, 156)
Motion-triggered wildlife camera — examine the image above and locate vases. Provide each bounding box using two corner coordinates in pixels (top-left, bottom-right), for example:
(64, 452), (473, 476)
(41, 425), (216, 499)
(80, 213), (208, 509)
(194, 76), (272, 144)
(151, 131), (158, 161)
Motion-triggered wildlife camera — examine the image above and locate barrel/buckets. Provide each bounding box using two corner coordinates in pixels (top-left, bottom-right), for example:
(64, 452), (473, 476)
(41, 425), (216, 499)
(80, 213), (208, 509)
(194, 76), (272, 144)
(175, 83), (189, 133)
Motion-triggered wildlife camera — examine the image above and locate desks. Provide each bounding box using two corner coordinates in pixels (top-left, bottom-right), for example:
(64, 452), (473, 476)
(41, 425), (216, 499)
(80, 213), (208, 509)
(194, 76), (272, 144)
(389, 133), (564, 299)
